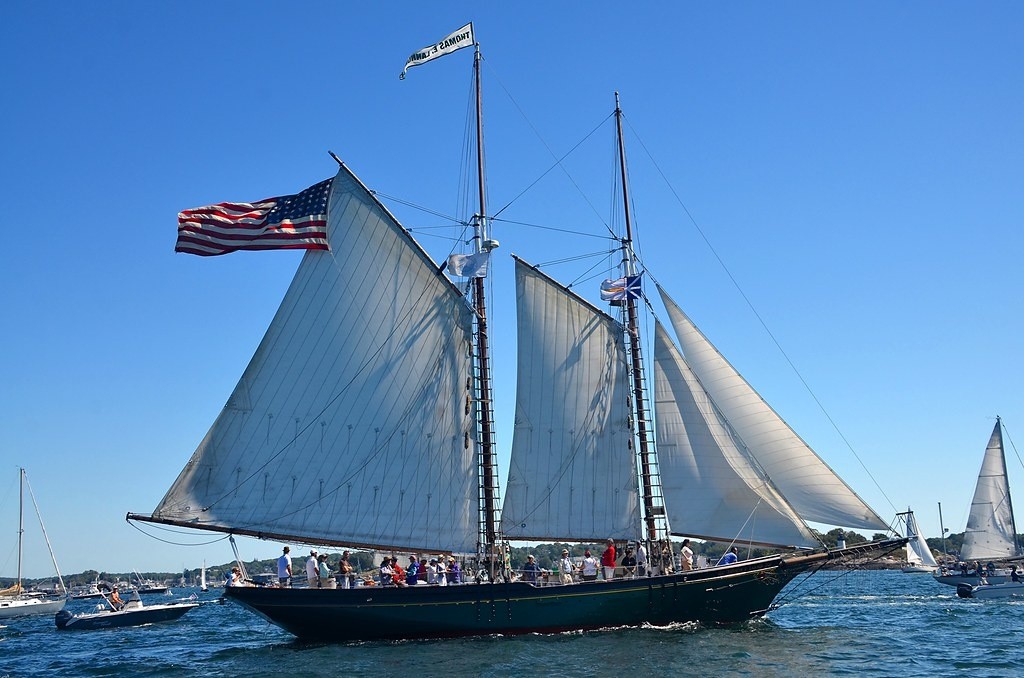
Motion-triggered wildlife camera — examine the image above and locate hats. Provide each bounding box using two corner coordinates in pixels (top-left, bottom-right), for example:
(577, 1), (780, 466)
(319, 554), (328, 558)
(384, 556), (392, 560)
(562, 549), (569, 554)
(391, 556), (398, 561)
(438, 554), (445, 559)
(607, 538), (614, 544)
(585, 550), (591, 556)
(310, 549), (318, 553)
(421, 559), (427, 562)
(283, 547), (290, 551)
(431, 559), (438, 563)
(447, 557), (455, 562)
(408, 555), (417, 560)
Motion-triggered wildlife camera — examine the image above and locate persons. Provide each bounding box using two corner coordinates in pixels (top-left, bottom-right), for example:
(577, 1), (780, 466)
(523, 555), (541, 586)
(558, 549), (573, 585)
(306, 550), (330, 587)
(621, 541), (647, 576)
(962, 561), (995, 574)
(680, 539), (693, 570)
(980, 571), (989, 585)
(601, 538), (616, 578)
(110, 586), (123, 611)
(578, 550), (600, 581)
(339, 551), (352, 589)
(1011, 566), (1024, 583)
(227, 567), (240, 585)
(277, 547), (292, 586)
(380, 554), (460, 585)
(720, 547), (737, 565)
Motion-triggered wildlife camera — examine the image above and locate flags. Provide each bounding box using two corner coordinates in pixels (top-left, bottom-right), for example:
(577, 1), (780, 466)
(174, 177), (334, 257)
(448, 251), (489, 278)
(600, 276), (641, 300)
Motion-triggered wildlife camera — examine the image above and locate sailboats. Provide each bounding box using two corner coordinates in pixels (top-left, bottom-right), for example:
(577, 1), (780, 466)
(126, 21), (920, 646)
(902, 414), (1024, 599)
(200, 559), (209, 592)
(0, 468), (68, 618)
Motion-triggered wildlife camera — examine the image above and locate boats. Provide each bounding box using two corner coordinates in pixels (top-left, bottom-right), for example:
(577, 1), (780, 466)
(70, 578), (168, 600)
(0, 584), (62, 598)
(54, 583), (199, 631)
